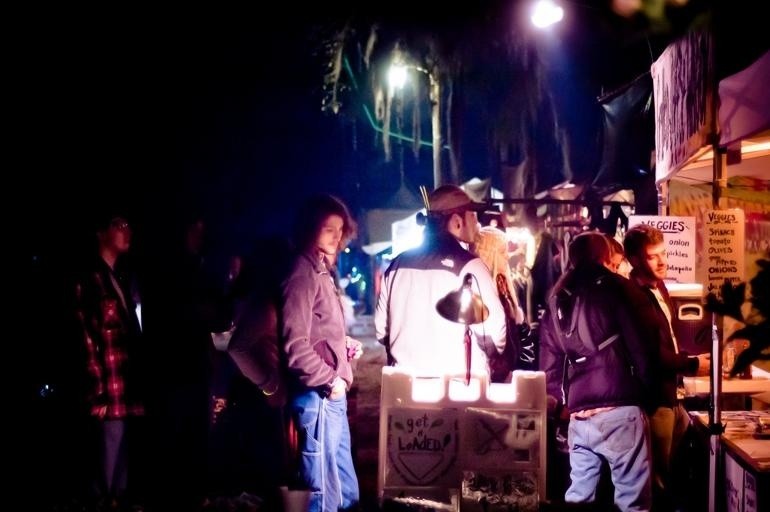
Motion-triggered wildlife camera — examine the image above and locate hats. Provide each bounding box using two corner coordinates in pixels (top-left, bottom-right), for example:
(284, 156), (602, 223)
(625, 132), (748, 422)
(427, 185), (482, 219)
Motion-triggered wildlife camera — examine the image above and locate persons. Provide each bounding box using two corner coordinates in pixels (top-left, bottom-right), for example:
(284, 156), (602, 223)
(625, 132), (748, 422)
(59, 184), (712, 512)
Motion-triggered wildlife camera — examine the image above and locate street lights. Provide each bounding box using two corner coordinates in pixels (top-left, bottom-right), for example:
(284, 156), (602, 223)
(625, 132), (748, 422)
(383, 57), (446, 193)
(523, 2), (569, 194)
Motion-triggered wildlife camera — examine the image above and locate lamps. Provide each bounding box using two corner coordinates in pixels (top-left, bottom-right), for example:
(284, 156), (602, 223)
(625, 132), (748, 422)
(432, 269), (494, 387)
